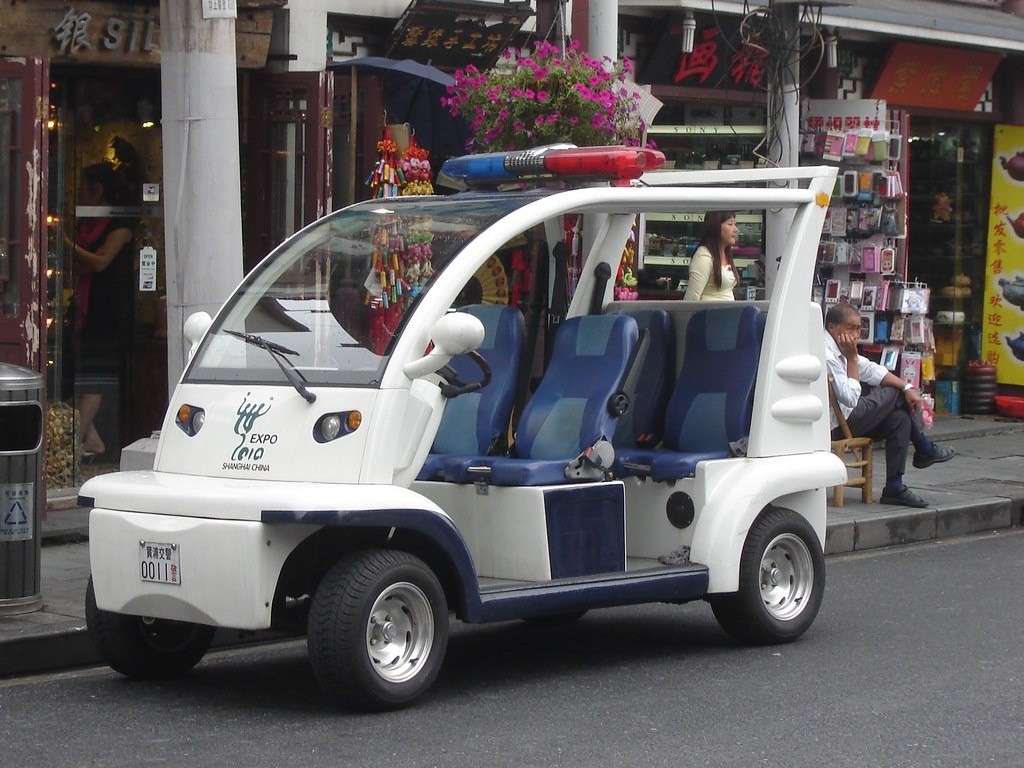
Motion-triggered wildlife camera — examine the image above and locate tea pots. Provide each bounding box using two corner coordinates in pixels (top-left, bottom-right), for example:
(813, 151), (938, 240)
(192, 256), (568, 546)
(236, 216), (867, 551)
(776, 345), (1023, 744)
(1000, 151), (1024, 181)
(999, 276), (1024, 306)
(1005, 332), (1024, 361)
(1006, 212), (1024, 238)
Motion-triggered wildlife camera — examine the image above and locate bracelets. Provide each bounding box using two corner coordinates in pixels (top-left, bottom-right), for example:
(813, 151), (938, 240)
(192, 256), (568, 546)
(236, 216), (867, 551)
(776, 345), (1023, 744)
(70, 242), (77, 250)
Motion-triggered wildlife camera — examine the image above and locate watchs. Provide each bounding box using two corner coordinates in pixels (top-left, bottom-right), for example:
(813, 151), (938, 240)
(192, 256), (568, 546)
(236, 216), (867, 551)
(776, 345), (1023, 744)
(903, 383), (914, 392)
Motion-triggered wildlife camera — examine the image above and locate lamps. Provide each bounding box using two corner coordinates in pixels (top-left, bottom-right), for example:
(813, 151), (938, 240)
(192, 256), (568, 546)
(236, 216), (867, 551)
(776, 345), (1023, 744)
(824, 26), (838, 68)
(681, 9), (696, 53)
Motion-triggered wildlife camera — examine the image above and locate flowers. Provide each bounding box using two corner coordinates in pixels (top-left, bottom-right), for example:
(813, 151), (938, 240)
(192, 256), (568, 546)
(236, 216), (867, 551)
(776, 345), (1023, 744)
(440, 37), (657, 150)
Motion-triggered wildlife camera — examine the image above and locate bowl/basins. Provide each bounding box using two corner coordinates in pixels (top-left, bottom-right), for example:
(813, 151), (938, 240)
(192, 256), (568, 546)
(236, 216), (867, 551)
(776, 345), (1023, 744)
(953, 273), (971, 287)
(937, 311), (965, 323)
(942, 286), (961, 297)
(994, 395), (1024, 418)
(962, 286), (972, 296)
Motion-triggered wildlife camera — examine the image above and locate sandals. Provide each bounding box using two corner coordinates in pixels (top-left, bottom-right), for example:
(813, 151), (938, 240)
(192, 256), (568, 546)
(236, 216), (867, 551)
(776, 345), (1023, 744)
(912, 440), (956, 468)
(880, 483), (929, 508)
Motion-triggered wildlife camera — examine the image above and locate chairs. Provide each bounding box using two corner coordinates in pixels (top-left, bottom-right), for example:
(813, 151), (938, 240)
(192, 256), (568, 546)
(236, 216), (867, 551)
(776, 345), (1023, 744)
(828, 381), (874, 508)
(415, 289), (547, 481)
(444, 313), (651, 487)
(614, 304), (766, 483)
(605, 308), (676, 449)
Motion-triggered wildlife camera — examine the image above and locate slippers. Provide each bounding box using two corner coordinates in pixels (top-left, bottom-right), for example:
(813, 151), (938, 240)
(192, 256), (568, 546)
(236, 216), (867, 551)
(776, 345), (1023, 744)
(83, 451), (95, 465)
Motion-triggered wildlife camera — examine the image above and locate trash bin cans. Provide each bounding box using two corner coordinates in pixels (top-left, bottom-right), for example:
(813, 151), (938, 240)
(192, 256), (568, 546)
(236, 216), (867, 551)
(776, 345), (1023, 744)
(0, 363), (47, 616)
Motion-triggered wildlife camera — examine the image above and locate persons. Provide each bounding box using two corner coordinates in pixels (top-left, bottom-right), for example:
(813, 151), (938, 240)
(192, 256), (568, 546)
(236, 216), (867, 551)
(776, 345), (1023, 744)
(683, 210), (740, 301)
(824, 304), (956, 508)
(46, 164), (135, 465)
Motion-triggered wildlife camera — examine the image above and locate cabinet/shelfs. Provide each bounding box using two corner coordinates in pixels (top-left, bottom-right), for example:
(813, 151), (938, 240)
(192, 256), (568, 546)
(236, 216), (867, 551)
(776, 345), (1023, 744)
(909, 148), (982, 330)
